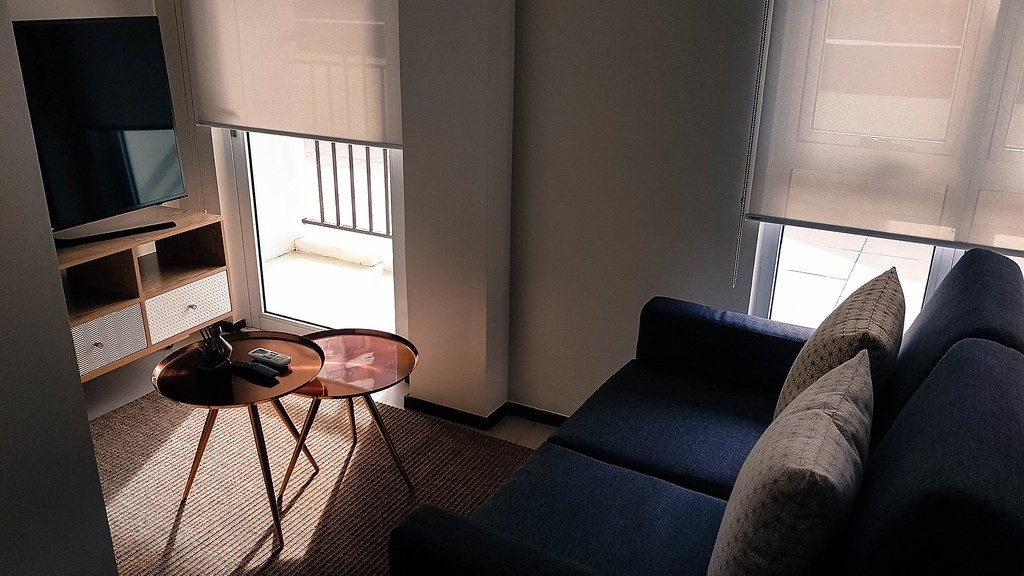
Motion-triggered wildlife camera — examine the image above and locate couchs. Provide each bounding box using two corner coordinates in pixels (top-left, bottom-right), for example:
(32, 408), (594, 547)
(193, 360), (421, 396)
(387, 247), (1024, 576)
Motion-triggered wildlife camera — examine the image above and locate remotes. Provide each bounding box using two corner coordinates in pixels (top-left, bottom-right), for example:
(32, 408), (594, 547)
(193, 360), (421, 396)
(232, 360), (279, 379)
(248, 348), (292, 367)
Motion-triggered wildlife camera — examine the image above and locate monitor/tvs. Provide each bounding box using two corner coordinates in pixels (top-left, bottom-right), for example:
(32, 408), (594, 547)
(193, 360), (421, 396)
(10, 16), (188, 246)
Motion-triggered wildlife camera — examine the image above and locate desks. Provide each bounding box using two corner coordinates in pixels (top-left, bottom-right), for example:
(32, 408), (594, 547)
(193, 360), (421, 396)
(151, 331), (325, 547)
(278, 328), (419, 499)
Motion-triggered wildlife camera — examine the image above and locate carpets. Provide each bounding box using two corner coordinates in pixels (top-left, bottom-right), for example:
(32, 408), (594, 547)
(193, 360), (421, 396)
(87, 389), (536, 576)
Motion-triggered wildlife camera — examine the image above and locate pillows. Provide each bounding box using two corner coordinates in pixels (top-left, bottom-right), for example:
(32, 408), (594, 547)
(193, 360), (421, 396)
(706, 349), (874, 576)
(772, 268), (905, 425)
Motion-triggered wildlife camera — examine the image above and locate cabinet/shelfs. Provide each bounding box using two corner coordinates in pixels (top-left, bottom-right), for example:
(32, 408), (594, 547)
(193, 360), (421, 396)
(53, 205), (235, 385)
